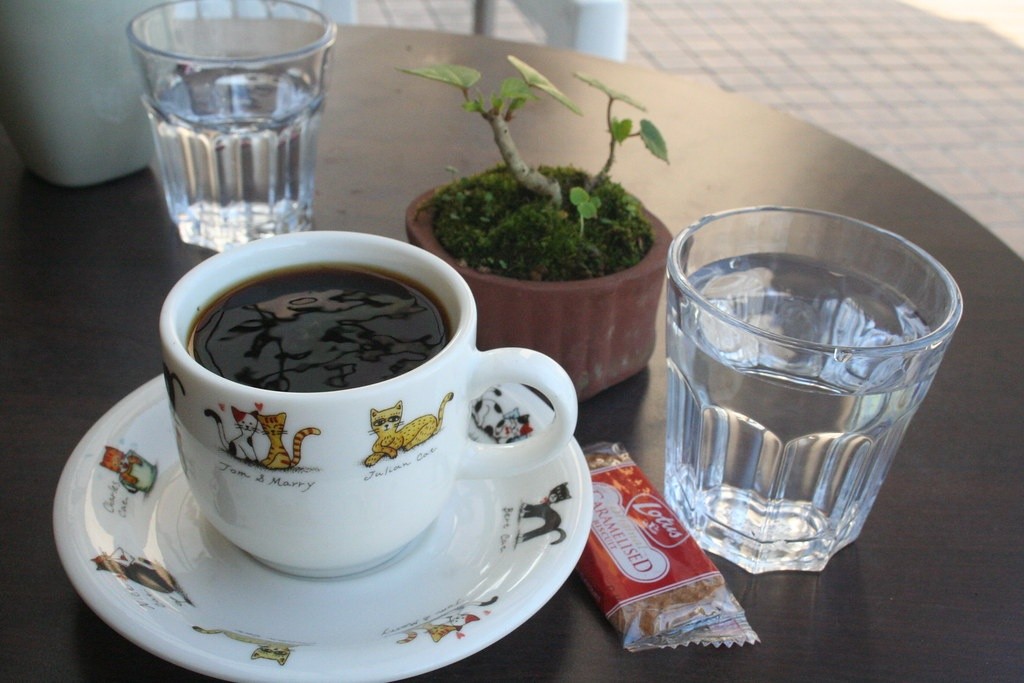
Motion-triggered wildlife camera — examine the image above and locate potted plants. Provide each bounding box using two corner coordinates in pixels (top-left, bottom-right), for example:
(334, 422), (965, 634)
(394, 53), (672, 403)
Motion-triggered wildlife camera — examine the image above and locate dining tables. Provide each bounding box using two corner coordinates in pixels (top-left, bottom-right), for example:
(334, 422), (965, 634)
(0, 18), (1024, 682)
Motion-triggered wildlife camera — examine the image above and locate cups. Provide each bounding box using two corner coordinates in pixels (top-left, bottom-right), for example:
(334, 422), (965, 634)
(663, 205), (963, 574)
(0, 0), (169, 187)
(160, 230), (579, 579)
(126, 0), (338, 254)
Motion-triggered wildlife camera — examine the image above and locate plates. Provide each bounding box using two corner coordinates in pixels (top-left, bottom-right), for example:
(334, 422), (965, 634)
(52, 370), (594, 683)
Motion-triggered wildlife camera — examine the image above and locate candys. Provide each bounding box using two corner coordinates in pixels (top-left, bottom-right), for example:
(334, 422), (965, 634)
(568, 441), (760, 652)
